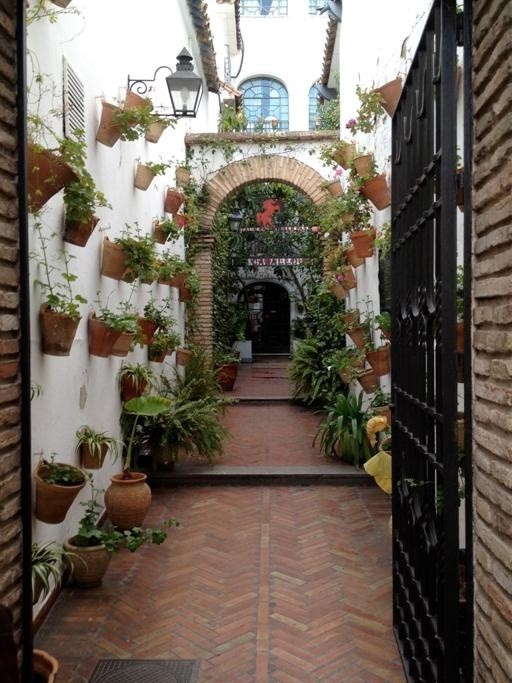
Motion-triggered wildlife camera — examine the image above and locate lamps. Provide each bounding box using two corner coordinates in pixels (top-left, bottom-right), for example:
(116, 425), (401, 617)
(127, 47), (203, 117)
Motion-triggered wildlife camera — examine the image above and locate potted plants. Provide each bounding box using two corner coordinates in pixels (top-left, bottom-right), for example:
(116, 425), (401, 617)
(26, 90), (240, 606)
(287, 75), (464, 463)
